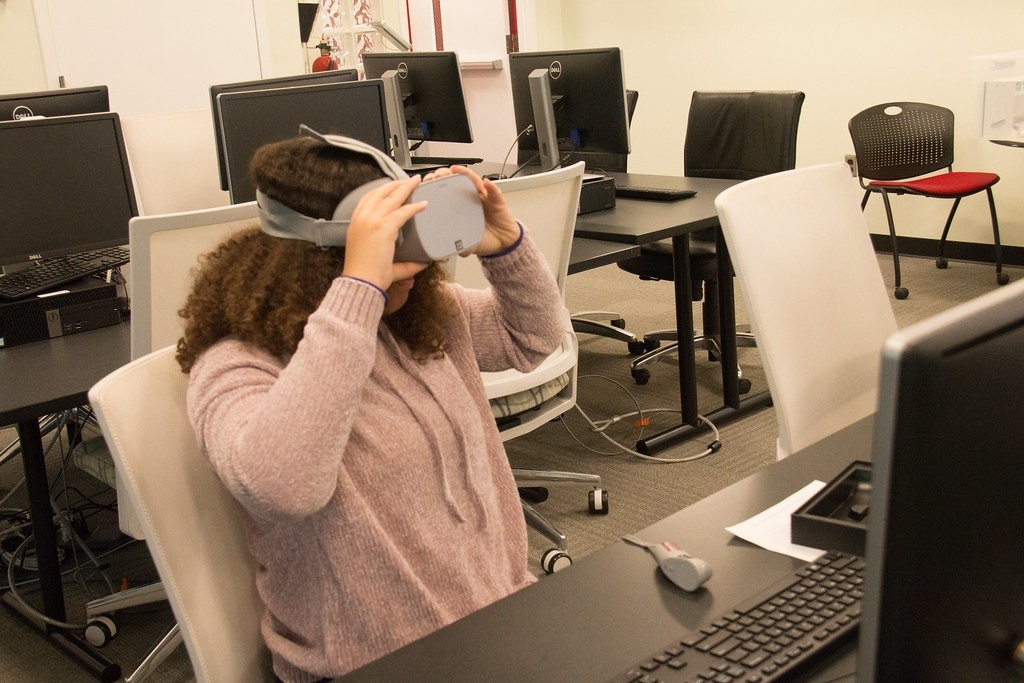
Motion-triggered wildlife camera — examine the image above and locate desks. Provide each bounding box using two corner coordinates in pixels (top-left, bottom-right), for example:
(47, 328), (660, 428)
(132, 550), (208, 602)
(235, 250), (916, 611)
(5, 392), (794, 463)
(327, 411), (877, 683)
(0, 167), (796, 683)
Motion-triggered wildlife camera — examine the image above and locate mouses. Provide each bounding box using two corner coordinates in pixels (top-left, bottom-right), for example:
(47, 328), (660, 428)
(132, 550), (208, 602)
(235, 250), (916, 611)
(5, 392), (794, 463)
(482, 174), (507, 181)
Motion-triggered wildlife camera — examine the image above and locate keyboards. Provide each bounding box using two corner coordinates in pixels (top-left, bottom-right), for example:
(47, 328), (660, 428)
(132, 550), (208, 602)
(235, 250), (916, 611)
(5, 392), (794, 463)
(410, 157), (484, 165)
(577, 176), (617, 216)
(0, 245), (132, 302)
(608, 546), (866, 683)
(615, 185), (697, 202)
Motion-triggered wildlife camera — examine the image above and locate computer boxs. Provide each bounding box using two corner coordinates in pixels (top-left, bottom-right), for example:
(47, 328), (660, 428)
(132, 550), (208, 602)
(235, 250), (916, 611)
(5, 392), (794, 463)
(0, 267), (130, 348)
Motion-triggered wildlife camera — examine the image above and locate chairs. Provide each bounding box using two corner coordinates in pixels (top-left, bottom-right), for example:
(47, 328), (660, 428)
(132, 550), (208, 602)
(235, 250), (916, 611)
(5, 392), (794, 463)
(84, 201), (264, 683)
(630, 90), (806, 392)
(433, 160), (609, 578)
(846, 102), (1010, 299)
(715, 163), (900, 465)
(85, 343), (281, 683)
(569, 88), (646, 355)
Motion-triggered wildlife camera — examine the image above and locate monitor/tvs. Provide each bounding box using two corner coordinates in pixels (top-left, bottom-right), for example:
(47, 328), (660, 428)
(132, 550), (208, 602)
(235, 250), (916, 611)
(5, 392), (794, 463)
(857, 277), (1024, 683)
(0, 85), (145, 276)
(208, 53), (476, 208)
(510, 49), (632, 182)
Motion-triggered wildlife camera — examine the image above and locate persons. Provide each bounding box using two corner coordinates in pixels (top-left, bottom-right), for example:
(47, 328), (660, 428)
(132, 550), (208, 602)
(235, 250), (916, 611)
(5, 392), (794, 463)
(178, 134), (565, 683)
